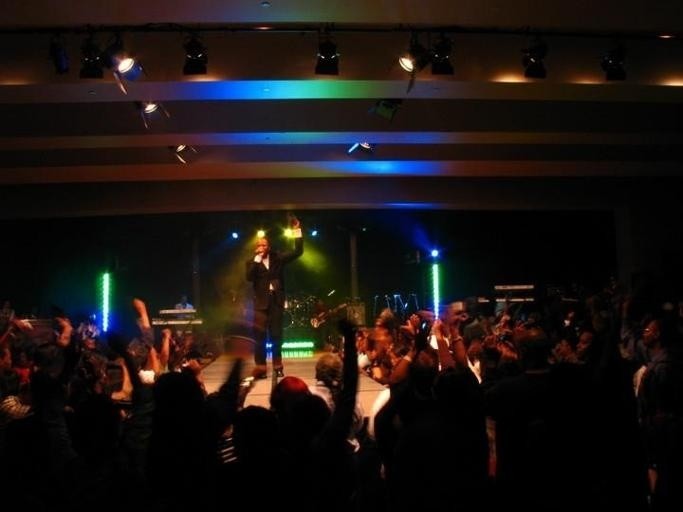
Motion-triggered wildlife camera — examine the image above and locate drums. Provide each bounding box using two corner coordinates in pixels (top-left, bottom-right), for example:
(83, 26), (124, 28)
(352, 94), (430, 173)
(283, 310), (294, 329)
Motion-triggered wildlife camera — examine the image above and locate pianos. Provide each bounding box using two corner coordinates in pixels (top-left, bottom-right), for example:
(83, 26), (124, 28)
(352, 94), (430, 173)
(151, 317), (202, 326)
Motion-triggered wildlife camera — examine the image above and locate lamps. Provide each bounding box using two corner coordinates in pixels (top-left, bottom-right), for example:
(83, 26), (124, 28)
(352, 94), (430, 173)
(399, 26), (456, 74)
(366, 100), (405, 122)
(176, 24), (209, 74)
(137, 103), (200, 165)
(43, 29), (143, 95)
(522, 46), (626, 81)
(314, 36), (338, 74)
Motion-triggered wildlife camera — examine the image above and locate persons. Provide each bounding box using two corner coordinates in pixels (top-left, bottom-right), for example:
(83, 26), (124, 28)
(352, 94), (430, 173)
(245, 217), (304, 380)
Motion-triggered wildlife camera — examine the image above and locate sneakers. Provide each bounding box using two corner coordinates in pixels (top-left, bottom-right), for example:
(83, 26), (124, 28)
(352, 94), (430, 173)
(252, 366), (284, 379)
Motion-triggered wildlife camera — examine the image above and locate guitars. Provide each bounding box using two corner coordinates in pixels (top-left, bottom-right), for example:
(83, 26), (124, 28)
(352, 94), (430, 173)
(310, 303), (348, 329)
